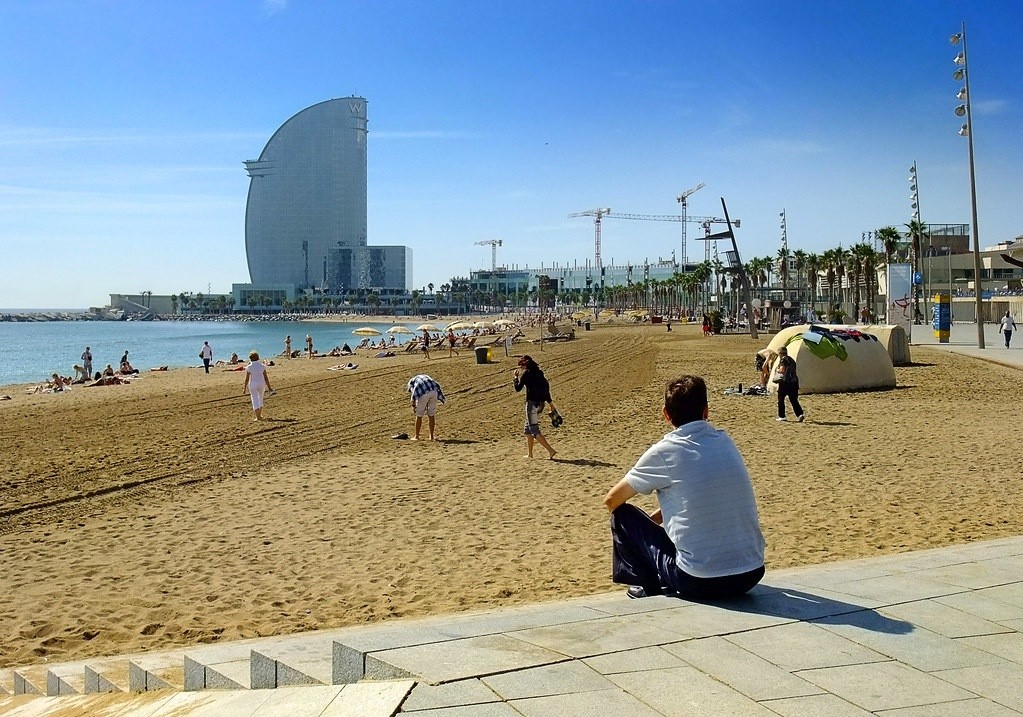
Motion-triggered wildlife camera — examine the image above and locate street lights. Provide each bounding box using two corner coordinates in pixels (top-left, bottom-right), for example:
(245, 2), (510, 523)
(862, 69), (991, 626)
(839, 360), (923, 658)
(779, 208), (789, 297)
(951, 21), (989, 350)
(909, 161), (936, 327)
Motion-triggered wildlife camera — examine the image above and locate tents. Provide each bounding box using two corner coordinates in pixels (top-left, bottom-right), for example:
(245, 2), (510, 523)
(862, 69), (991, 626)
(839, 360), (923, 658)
(757, 324), (911, 395)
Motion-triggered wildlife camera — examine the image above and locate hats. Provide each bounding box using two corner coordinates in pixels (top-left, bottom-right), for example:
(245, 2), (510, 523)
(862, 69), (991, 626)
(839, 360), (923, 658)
(232, 353), (236, 356)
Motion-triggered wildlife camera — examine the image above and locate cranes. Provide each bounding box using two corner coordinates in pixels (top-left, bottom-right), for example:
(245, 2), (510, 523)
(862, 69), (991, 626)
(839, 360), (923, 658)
(567, 206), (741, 271)
(676, 182), (706, 273)
(475, 240), (502, 271)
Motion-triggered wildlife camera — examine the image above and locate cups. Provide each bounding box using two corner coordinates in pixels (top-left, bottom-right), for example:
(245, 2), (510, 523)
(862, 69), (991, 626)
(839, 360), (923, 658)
(739, 383), (742, 392)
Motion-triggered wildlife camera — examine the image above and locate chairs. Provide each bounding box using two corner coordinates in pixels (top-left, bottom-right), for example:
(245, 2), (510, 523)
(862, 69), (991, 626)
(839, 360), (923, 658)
(401, 332), (574, 352)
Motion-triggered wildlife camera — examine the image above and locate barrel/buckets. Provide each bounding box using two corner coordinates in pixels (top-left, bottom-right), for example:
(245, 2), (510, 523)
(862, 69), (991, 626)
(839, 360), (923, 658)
(476, 348), (488, 364)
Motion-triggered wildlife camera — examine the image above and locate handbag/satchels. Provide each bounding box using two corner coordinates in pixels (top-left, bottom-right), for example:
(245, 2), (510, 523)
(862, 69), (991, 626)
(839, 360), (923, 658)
(772, 366), (789, 383)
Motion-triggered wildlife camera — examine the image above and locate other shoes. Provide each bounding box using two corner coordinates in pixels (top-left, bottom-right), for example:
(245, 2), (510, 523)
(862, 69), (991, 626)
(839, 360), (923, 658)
(549, 410), (563, 428)
(626, 586), (647, 599)
(267, 389), (277, 396)
(391, 432), (408, 439)
(798, 414), (804, 422)
(1006, 346), (1009, 349)
(776, 416), (787, 421)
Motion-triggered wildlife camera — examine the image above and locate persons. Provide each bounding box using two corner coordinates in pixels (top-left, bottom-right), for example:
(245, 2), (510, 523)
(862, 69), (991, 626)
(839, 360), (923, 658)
(241, 351), (274, 422)
(604, 376), (766, 602)
(756, 345), (806, 423)
(998, 310), (1017, 349)
(199, 341), (238, 375)
(807, 306), (880, 325)
(332, 363), (353, 368)
(781, 318), (804, 330)
(931, 305), (935, 321)
(263, 359), (275, 366)
(665, 315), (672, 333)
(407, 374), (445, 441)
(514, 355), (557, 460)
(284, 331), (497, 359)
(702, 313), (713, 336)
(752, 307), (762, 330)
(34, 341), (135, 390)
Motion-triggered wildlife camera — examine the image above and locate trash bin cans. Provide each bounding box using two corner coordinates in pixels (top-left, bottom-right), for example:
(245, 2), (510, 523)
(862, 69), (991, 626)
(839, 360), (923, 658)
(474, 348), (488, 364)
(482, 346), (492, 363)
(577, 320), (581, 326)
(585, 322), (590, 330)
(652, 317), (662, 323)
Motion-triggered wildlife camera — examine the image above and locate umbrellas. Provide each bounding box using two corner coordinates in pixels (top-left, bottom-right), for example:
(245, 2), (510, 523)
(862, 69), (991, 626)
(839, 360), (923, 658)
(386, 325), (412, 345)
(475, 320), (515, 347)
(352, 327), (382, 338)
(416, 324), (442, 345)
(573, 309), (650, 326)
(443, 319), (475, 349)
